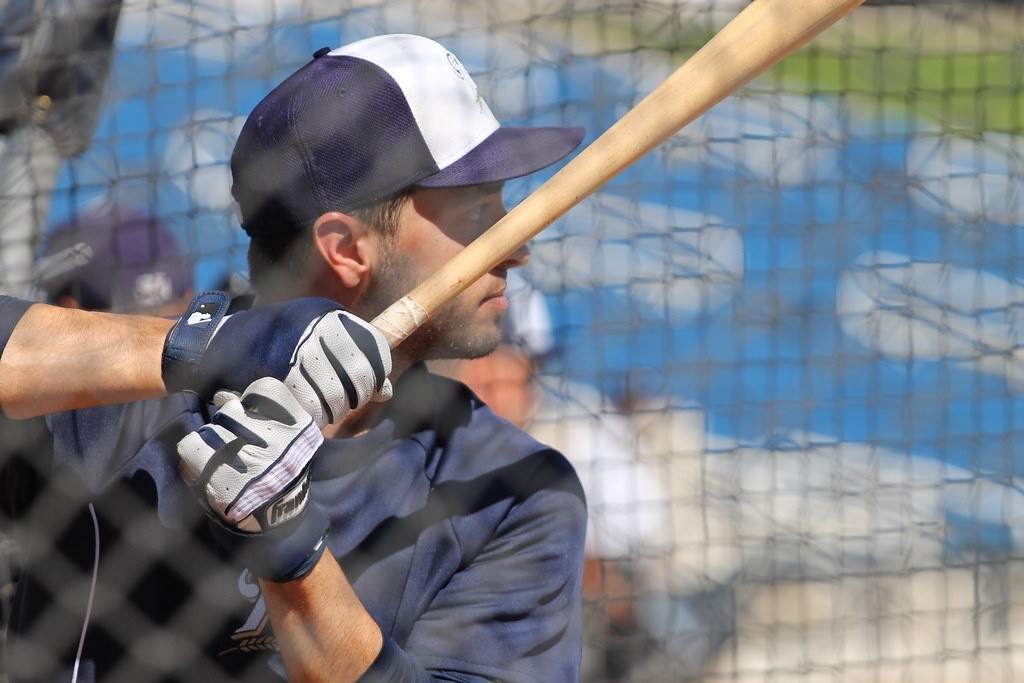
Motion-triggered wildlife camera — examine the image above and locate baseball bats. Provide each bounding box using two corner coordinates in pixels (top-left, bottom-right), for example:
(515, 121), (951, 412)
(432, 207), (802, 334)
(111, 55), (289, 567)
(178, 1), (869, 493)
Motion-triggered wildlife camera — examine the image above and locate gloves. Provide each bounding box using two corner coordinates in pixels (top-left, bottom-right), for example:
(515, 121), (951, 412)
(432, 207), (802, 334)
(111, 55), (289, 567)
(160, 291), (393, 431)
(176, 375), (331, 583)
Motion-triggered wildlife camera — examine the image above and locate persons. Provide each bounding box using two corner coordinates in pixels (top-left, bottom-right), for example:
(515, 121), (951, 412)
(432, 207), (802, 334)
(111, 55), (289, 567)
(425, 269), (677, 683)
(0, 0), (122, 306)
(0, 33), (586, 683)
(48, 195), (194, 322)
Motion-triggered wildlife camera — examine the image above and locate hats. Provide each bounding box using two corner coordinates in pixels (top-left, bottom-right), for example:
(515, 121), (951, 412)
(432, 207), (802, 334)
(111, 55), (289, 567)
(41, 206), (195, 312)
(231, 34), (586, 238)
